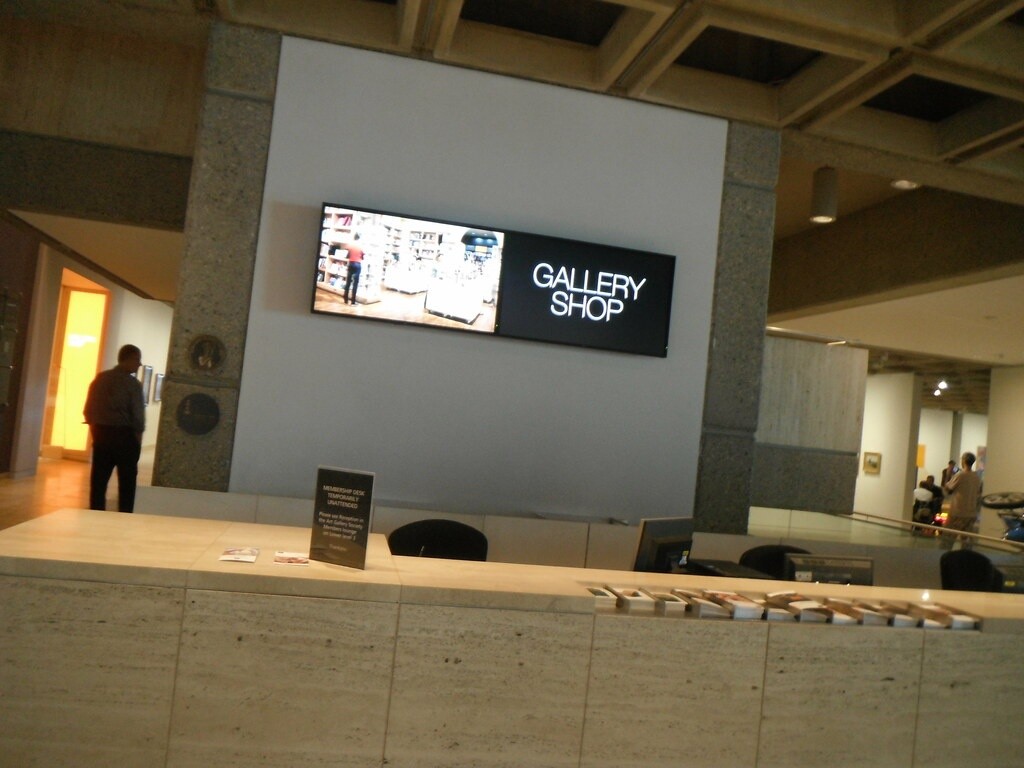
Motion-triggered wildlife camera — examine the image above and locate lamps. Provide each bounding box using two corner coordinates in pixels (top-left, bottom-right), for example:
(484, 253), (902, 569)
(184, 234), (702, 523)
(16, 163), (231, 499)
(808, 166), (839, 225)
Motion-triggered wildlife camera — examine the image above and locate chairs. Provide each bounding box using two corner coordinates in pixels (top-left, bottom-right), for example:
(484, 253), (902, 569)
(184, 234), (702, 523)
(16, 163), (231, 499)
(739, 545), (811, 570)
(940, 548), (993, 591)
(387, 519), (489, 561)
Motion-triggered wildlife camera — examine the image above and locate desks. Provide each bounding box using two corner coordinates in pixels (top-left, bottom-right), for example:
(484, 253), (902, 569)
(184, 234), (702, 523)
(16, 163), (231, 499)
(679, 559), (770, 581)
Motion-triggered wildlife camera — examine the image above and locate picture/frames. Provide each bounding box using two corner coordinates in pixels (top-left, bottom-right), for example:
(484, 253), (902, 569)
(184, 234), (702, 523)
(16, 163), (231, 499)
(141, 365), (163, 404)
(863, 452), (881, 475)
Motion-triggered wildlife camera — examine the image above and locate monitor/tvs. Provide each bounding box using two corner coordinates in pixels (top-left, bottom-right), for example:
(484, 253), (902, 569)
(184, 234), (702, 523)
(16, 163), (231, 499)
(310, 202), (676, 359)
(633, 516), (697, 573)
(784, 554), (873, 586)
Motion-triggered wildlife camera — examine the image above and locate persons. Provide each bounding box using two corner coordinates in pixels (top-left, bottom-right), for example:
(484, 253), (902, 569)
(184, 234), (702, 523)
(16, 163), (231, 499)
(940, 452), (983, 551)
(84, 344), (145, 515)
(344, 233), (364, 306)
(941, 460), (956, 487)
(913, 475), (942, 519)
